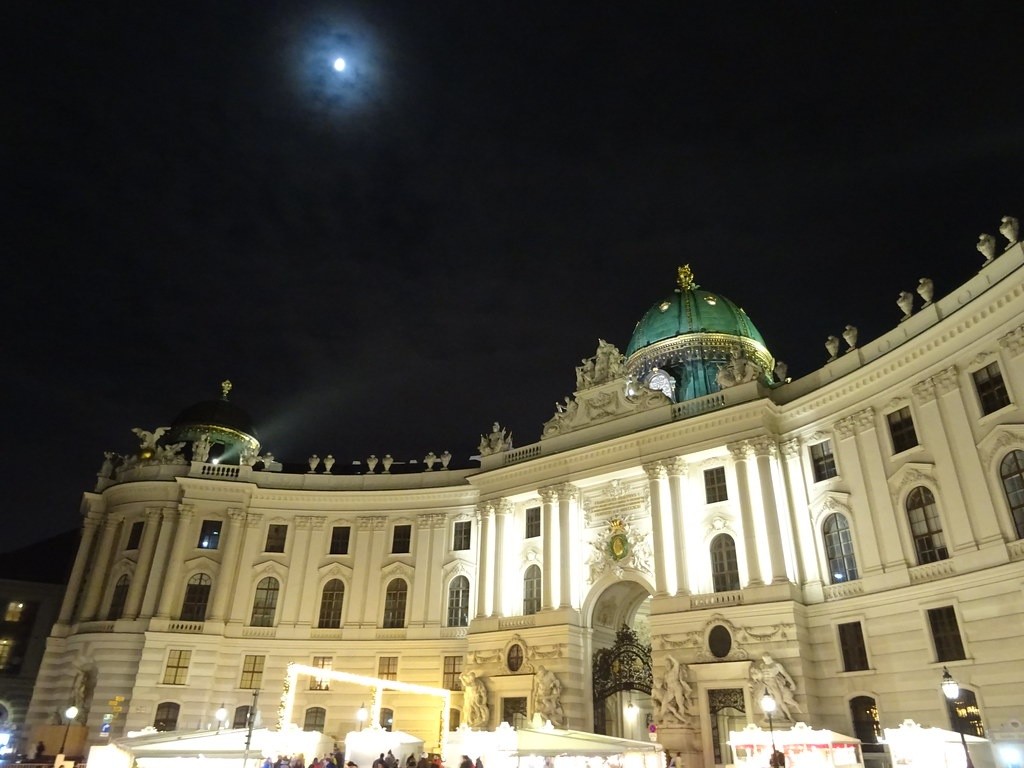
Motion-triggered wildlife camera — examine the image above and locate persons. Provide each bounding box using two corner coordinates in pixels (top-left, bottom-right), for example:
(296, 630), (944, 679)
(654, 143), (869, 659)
(666, 749), (685, 768)
(543, 397), (577, 426)
(192, 433), (209, 462)
(35, 742), (46, 757)
(483, 423), (513, 452)
(459, 672), (490, 725)
(263, 744), (483, 768)
(533, 665), (561, 726)
(749, 652), (799, 722)
(651, 656), (692, 723)
(576, 339), (623, 386)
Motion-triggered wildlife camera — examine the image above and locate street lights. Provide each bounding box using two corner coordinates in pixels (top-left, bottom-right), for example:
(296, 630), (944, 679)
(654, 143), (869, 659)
(761, 687), (779, 768)
(357, 702), (367, 731)
(59, 700), (78, 754)
(941, 666), (975, 768)
(215, 703), (228, 734)
